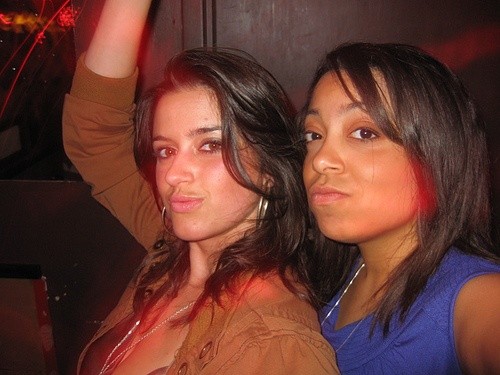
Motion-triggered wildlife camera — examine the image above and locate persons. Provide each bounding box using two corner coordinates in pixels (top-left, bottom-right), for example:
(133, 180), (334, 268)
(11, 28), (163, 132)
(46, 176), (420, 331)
(299, 42), (499, 375)
(60, 1), (341, 375)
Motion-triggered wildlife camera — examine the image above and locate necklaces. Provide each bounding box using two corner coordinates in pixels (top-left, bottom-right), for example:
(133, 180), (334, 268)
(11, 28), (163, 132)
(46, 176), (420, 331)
(98, 295), (202, 374)
(319, 264), (394, 355)
